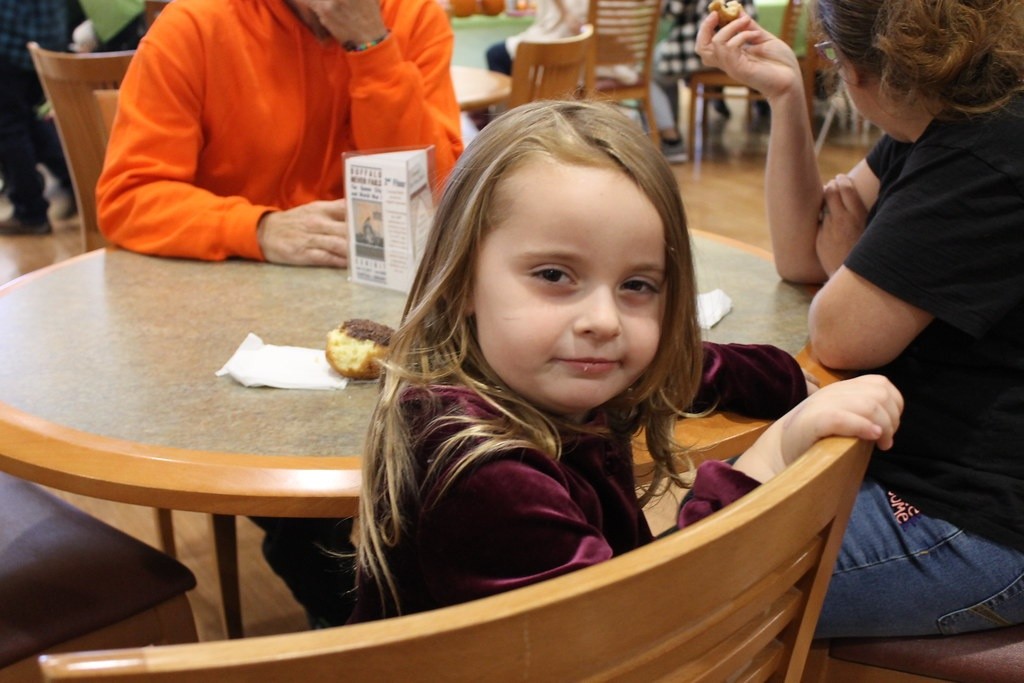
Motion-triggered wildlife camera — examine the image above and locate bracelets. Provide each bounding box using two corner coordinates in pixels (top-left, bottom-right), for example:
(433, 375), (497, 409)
(341, 28), (392, 51)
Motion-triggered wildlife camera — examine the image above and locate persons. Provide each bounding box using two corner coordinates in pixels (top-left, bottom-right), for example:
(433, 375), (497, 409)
(696, 0), (1024, 640)
(450, 0), (590, 75)
(0, 0), (103, 235)
(350, 102), (903, 622)
(649, 0), (757, 162)
(92, 0), (463, 267)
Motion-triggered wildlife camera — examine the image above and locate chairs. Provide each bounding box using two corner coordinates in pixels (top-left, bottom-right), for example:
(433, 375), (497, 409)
(28, 44), (246, 640)
(0, 436), (1024, 682)
(508, 0), (839, 166)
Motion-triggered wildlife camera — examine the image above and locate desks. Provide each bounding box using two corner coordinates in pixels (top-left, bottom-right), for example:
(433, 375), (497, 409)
(0, 230), (851, 640)
(453, 65), (510, 112)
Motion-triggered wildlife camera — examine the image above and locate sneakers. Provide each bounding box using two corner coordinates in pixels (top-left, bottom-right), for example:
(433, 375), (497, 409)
(1, 214), (52, 234)
(658, 140), (689, 162)
(55, 188), (78, 220)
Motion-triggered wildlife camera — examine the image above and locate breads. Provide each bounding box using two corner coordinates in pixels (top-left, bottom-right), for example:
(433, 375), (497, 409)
(324, 318), (397, 380)
(707, 0), (741, 26)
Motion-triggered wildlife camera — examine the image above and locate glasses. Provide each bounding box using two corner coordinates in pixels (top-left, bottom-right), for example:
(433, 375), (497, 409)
(813, 41), (840, 62)
